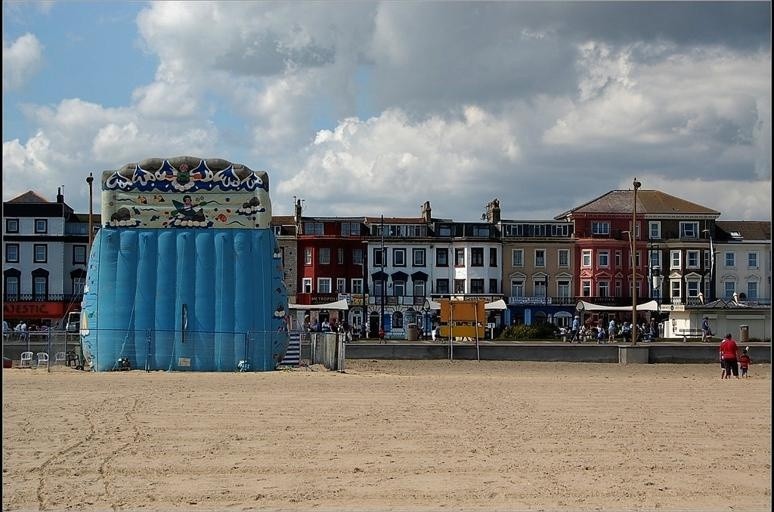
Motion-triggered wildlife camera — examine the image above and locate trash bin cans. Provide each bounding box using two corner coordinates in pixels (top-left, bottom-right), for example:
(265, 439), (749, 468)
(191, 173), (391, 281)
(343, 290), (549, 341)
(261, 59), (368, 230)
(739, 325), (750, 342)
(407, 323), (418, 341)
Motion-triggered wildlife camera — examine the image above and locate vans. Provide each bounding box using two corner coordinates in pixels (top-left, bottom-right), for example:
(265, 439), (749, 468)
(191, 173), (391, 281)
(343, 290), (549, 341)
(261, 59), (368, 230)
(65, 312), (80, 332)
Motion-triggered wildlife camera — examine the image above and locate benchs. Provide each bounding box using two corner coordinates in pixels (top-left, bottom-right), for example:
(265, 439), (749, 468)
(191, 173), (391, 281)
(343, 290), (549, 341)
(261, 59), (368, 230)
(675, 334), (714, 343)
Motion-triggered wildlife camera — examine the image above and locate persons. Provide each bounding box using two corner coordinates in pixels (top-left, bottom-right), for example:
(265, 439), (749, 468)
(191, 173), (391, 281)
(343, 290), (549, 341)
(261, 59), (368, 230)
(302, 318), (367, 343)
(702, 317), (714, 342)
(559, 315), (656, 344)
(432, 323), (437, 341)
(719, 333), (750, 379)
(3, 320), (48, 343)
(379, 325), (387, 344)
